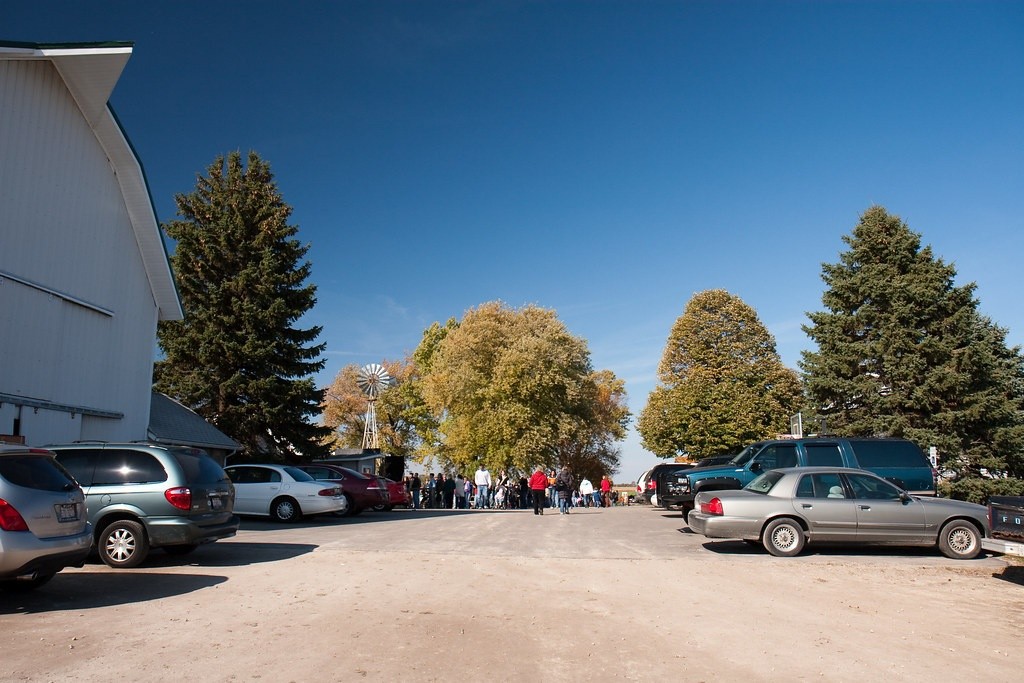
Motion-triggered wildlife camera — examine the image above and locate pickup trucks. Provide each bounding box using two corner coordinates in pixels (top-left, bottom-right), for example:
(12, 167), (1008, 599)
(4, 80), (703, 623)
(981, 493), (1024, 542)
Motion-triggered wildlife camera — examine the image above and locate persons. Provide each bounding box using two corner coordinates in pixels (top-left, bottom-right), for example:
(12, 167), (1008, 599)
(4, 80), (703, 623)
(403, 463), (618, 515)
(556, 466), (574, 514)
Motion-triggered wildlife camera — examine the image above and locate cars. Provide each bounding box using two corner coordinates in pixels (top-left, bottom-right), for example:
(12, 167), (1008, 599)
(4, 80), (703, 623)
(283, 464), (409, 523)
(221, 465), (347, 525)
(689, 464), (990, 559)
(0, 442), (95, 596)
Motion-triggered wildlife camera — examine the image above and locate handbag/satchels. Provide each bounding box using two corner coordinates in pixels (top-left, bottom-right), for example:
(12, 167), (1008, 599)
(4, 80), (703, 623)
(555, 473), (566, 492)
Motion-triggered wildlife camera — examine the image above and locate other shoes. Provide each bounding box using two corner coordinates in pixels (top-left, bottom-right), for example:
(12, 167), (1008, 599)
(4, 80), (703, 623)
(561, 511), (564, 514)
(479, 506), (482, 509)
(565, 509), (569, 514)
(484, 505), (488, 508)
(539, 509), (543, 516)
(534, 507), (539, 515)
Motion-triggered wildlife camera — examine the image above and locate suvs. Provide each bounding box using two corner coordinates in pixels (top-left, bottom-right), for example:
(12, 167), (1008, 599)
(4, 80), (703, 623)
(659, 439), (937, 524)
(636, 455), (734, 510)
(32, 439), (241, 567)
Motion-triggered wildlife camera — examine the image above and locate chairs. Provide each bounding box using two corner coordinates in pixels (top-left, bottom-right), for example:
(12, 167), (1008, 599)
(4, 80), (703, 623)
(827, 486), (844, 499)
